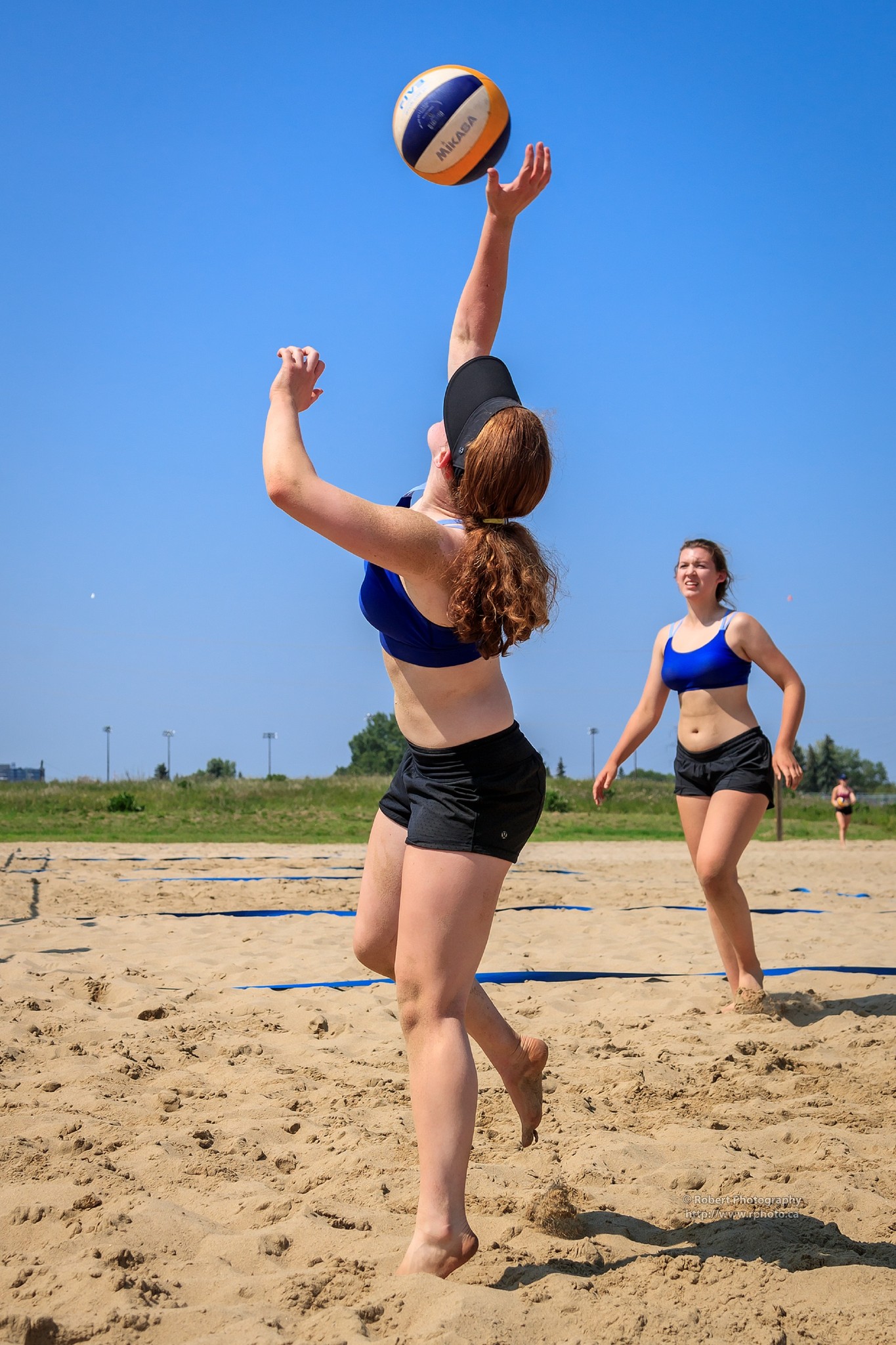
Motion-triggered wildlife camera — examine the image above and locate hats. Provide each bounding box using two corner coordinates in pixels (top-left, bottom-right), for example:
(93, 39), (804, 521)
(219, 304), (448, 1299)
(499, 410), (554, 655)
(443, 355), (530, 488)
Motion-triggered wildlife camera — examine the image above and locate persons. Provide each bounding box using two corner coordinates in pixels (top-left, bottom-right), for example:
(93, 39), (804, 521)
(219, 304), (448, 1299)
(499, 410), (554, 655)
(831, 774), (856, 842)
(592, 539), (805, 1009)
(261, 144), (564, 1279)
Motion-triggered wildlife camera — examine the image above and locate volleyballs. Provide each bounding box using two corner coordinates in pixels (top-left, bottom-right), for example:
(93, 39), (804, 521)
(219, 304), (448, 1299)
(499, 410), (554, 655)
(392, 64), (511, 186)
(836, 797), (846, 807)
